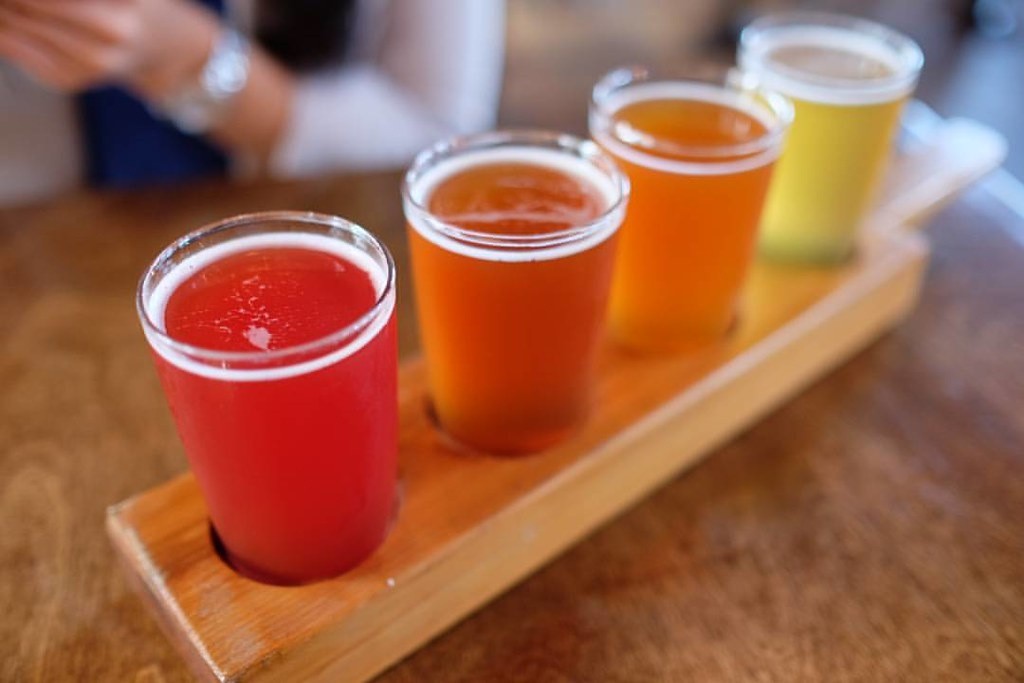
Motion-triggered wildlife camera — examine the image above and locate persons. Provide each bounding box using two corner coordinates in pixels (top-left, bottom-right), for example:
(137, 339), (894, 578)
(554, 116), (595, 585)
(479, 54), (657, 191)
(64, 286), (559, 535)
(0, 0), (502, 199)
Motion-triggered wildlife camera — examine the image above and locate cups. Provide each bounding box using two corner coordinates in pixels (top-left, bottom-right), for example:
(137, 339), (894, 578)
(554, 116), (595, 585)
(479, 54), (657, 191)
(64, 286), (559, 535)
(727, 11), (925, 270)
(400, 127), (632, 463)
(586, 59), (798, 363)
(134, 210), (400, 589)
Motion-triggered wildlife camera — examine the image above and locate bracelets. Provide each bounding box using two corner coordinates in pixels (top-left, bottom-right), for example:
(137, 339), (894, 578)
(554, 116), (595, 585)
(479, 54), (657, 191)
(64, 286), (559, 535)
(145, 17), (249, 135)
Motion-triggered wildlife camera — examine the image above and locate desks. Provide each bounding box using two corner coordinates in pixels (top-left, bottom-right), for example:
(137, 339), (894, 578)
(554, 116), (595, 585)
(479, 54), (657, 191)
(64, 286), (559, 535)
(0, 0), (1024, 683)
